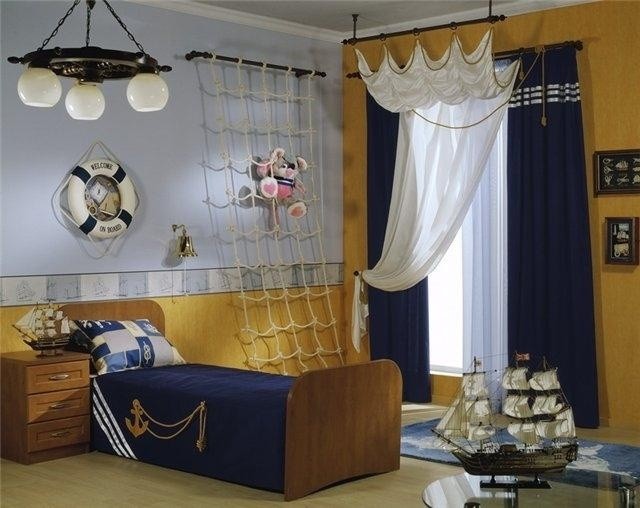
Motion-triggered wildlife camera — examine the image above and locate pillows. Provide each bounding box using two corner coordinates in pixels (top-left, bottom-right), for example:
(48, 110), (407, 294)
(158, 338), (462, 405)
(67, 319), (186, 376)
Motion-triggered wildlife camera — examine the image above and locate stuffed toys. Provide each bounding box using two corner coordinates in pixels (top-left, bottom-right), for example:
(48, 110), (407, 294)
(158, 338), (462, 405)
(255, 147), (309, 218)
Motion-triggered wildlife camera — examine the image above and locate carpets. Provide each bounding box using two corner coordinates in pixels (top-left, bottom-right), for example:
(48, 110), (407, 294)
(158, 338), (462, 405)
(400, 418), (640, 491)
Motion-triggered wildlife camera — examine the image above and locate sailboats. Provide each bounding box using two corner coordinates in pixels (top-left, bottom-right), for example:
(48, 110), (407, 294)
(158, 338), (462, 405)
(12, 302), (80, 358)
(430, 349), (579, 489)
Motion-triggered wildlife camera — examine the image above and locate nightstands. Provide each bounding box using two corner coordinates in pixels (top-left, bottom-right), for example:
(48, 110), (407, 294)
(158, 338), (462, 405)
(0, 350), (90, 465)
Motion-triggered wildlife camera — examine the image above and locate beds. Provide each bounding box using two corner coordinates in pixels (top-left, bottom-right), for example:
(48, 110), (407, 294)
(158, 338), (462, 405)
(51, 299), (404, 501)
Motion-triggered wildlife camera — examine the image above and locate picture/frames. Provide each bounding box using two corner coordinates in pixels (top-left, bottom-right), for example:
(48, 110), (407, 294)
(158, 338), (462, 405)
(604, 217), (638, 265)
(594, 149), (640, 198)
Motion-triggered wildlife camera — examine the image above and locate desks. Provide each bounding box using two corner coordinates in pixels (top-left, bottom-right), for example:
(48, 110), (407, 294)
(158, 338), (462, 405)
(422, 467), (640, 508)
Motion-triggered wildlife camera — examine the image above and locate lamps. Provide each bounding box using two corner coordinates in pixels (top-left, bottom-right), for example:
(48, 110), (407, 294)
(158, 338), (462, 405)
(7, 0), (172, 120)
(172, 225), (197, 258)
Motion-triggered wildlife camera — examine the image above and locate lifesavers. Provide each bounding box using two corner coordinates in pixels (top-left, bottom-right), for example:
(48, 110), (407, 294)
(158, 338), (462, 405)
(68, 157), (135, 238)
